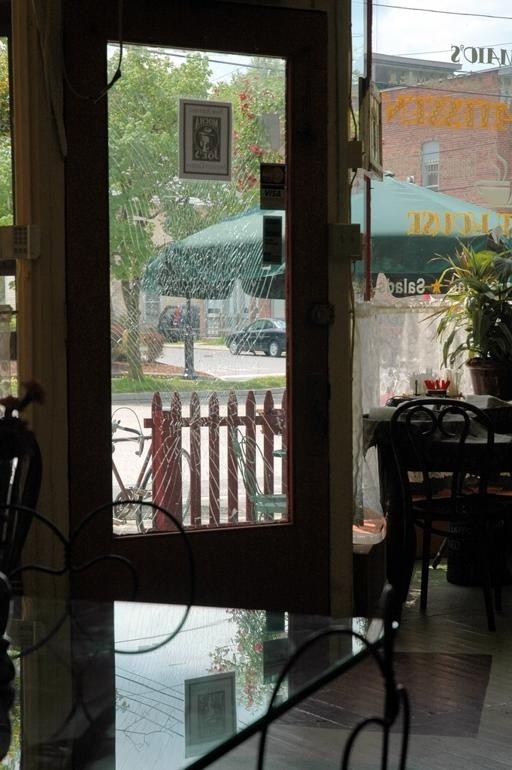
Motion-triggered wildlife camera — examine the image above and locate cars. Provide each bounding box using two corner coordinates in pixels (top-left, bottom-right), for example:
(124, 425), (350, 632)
(222, 314), (286, 361)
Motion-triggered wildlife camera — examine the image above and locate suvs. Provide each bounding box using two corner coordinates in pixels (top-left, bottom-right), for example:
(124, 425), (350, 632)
(158, 301), (200, 343)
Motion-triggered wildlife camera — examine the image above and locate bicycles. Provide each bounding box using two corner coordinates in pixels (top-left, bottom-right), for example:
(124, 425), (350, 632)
(111, 413), (193, 538)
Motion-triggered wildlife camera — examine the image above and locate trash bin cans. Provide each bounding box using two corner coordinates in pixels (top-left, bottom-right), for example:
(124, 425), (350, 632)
(447, 494), (512, 585)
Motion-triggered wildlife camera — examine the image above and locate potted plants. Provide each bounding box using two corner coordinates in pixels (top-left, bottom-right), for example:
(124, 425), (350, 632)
(418, 236), (512, 401)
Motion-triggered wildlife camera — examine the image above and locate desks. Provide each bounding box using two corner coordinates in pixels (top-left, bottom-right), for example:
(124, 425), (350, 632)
(362, 394), (511, 526)
(4, 584), (403, 768)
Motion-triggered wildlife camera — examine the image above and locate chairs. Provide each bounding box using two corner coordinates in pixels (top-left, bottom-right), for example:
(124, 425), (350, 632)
(250, 614), (416, 768)
(1, 500), (196, 608)
(385, 396), (499, 620)
